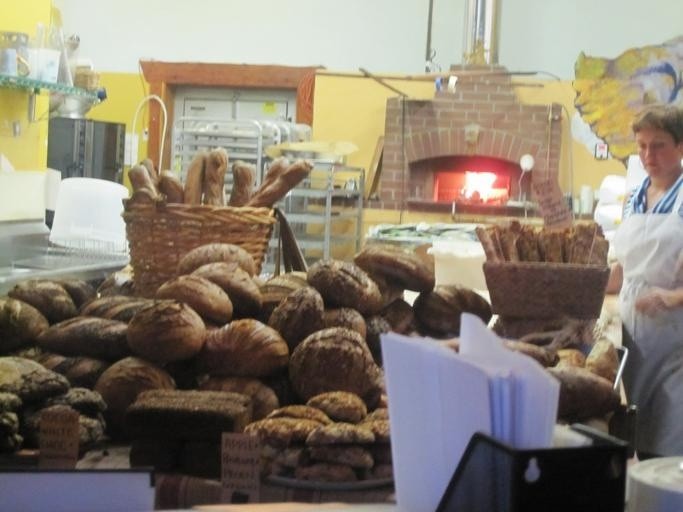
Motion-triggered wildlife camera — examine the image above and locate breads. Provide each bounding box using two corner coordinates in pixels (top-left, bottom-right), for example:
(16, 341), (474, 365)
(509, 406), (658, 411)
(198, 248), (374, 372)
(476, 220), (608, 266)
(1, 245), (622, 482)
(128, 148), (314, 210)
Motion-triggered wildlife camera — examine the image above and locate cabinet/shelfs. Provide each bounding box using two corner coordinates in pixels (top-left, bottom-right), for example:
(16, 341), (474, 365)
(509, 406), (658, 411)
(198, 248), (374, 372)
(261, 159), (366, 270)
(171, 117), (312, 269)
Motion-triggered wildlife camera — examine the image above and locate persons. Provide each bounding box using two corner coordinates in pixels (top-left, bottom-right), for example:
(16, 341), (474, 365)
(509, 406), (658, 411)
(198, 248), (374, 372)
(607, 104), (682, 463)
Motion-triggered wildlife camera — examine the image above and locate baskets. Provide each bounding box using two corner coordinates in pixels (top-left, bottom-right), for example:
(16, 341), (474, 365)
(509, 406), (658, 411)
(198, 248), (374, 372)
(116, 199), (277, 294)
(480, 259), (611, 322)
